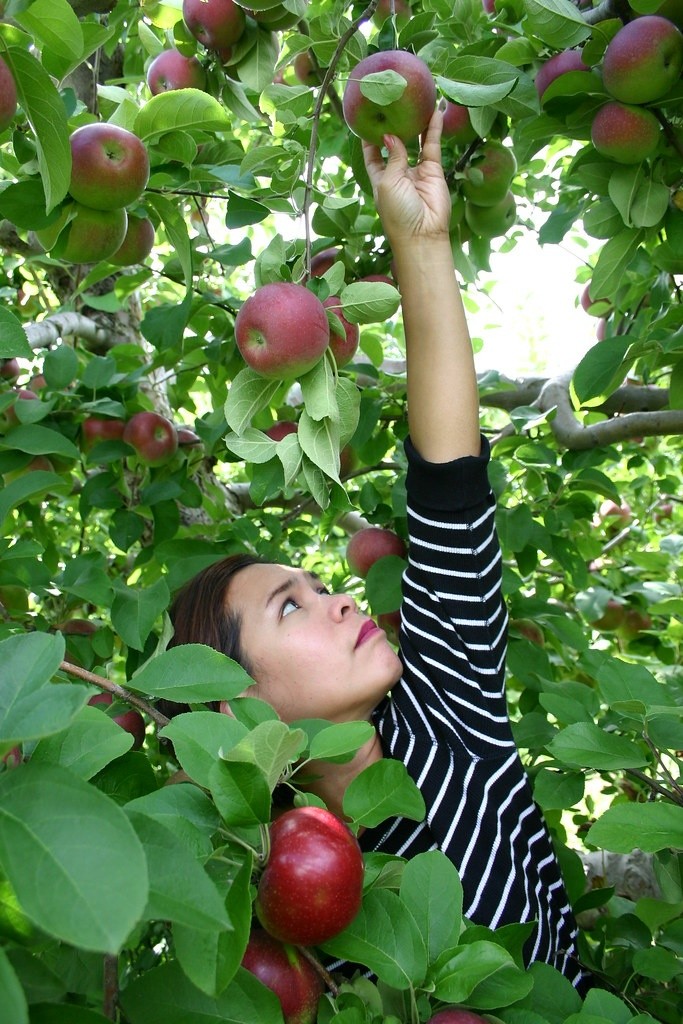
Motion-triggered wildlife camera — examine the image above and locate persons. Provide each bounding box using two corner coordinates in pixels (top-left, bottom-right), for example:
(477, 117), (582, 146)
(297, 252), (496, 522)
(144, 111), (584, 1024)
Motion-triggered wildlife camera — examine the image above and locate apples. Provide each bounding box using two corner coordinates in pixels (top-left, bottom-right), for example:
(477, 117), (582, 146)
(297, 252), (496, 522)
(378, 609), (401, 627)
(88, 692), (145, 751)
(253, 804), (365, 945)
(64, 619), (95, 661)
(625, 610), (650, 640)
(240, 930), (325, 1024)
(600, 498), (630, 523)
(512, 619), (544, 646)
(0, 1), (683, 481)
(655, 505), (672, 526)
(428, 1010), (488, 1024)
(346, 528), (407, 578)
(590, 600), (623, 630)
